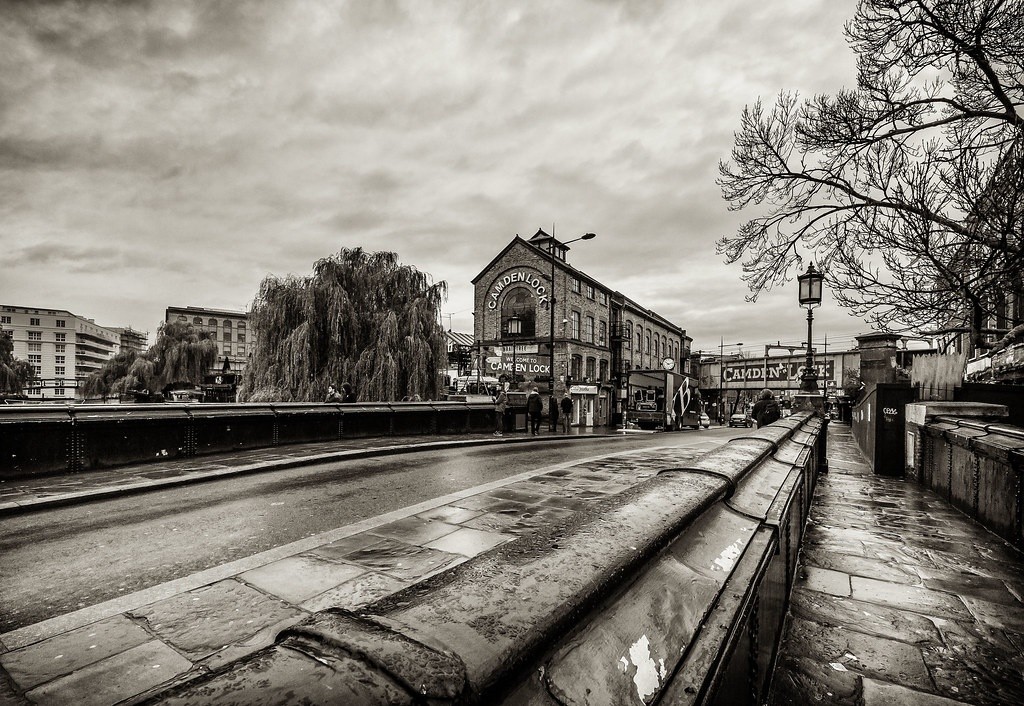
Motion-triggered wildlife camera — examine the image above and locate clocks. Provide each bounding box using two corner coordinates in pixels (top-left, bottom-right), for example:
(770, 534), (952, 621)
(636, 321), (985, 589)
(663, 357), (675, 370)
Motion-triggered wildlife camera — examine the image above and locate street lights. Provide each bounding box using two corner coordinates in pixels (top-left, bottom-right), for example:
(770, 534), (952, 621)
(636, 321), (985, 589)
(547, 222), (597, 429)
(507, 311), (523, 392)
(718, 337), (743, 425)
(791, 261), (824, 394)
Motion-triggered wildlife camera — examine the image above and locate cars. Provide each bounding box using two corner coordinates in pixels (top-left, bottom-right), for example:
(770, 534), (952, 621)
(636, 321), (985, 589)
(698, 413), (711, 428)
(729, 413), (754, 428)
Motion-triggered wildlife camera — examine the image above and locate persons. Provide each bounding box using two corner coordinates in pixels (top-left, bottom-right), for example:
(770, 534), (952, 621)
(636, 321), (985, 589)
(751, 389), (781, 430)
(560, 393), (574, 434)
(527, 386), (544, 436)
(322, 383), (358, 403)
(402, 393), (433, 403)
(548, 395), (559, 432)
(491, 382), (507, 437)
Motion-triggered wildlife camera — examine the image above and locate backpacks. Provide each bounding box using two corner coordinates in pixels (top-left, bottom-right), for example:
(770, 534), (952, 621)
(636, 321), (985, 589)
(761, 399), (779, 425)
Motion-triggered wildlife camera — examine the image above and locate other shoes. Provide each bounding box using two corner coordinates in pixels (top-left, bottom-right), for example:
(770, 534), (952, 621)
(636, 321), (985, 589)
(563, 432), (566, 434)
(492, 431), (503, 437)
(532, 431), (539, 437)
(567, 432), (570, 435)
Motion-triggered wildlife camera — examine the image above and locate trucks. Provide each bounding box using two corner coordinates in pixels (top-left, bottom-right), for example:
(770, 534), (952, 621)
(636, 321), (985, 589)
(626, 370), (702, 430)
